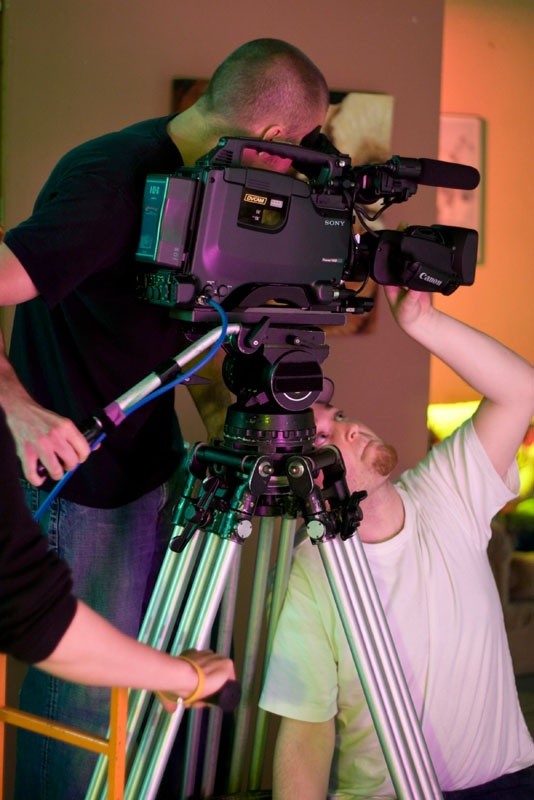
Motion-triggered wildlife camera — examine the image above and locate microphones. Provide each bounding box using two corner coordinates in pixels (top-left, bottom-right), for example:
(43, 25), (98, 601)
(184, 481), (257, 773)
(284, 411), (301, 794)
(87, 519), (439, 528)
(386, 156), (480, 190)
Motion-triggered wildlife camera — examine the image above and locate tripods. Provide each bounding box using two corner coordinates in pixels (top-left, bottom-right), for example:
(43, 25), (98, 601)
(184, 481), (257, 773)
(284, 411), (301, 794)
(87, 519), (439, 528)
(87, 397), (446, 800)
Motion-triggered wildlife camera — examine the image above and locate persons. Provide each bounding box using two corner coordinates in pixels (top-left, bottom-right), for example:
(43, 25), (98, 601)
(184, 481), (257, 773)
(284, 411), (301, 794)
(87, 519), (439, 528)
(254, 273), (534, 800)
(0, 35), (333, 703)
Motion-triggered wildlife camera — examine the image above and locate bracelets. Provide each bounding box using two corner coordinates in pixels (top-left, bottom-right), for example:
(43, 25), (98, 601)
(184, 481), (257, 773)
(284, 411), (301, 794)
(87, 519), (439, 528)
(156, 655), (204, 703)
